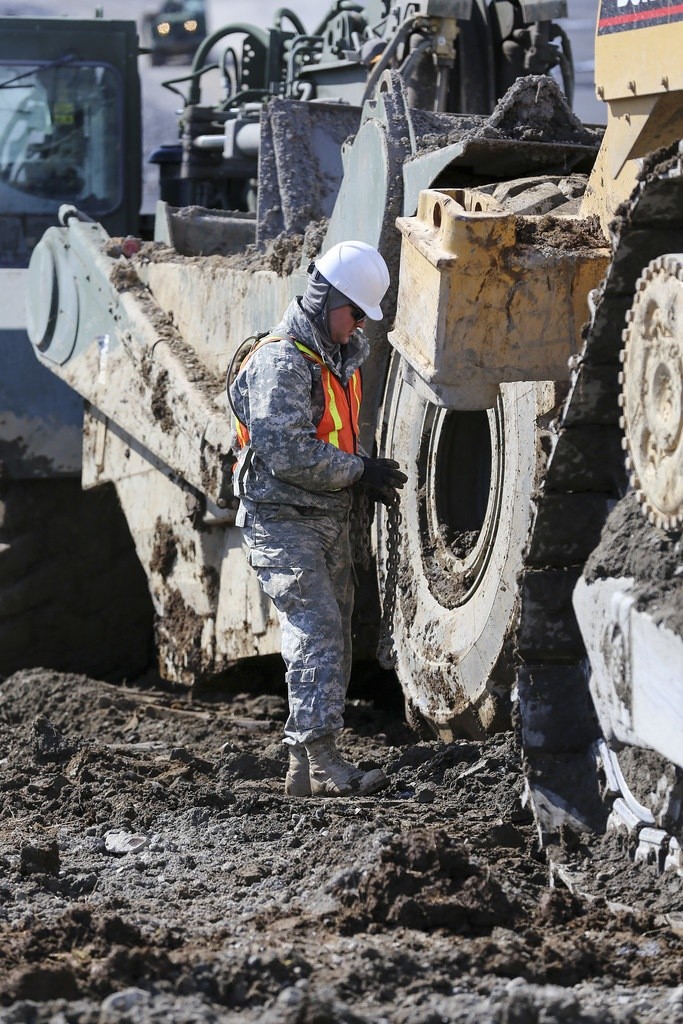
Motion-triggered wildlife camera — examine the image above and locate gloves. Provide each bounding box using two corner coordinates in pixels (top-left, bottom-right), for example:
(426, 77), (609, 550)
(358, 458), (408, 508)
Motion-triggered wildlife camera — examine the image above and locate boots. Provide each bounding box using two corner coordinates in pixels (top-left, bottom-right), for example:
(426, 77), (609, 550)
(285, 742), (312, 796)
(305, 733), (391, 797)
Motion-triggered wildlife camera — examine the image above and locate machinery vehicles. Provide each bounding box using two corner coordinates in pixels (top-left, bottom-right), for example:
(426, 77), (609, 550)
(25, 1), (682, 940)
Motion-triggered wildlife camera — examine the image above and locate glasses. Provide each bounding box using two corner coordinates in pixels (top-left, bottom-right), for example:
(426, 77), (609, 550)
(347, 304), (368, 322)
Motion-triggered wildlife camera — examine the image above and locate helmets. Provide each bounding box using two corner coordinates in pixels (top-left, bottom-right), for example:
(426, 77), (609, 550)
(315, 241), (391, 321)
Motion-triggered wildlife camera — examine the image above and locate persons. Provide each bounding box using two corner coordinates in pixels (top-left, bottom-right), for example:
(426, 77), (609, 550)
(231, 240), (407, 798)
